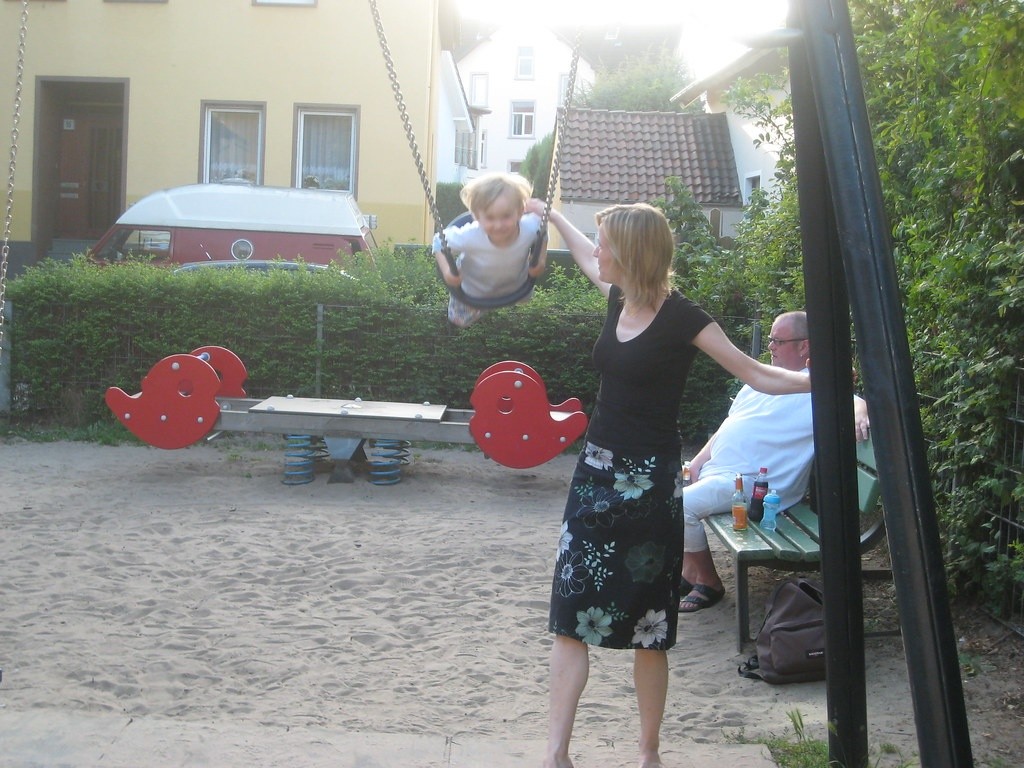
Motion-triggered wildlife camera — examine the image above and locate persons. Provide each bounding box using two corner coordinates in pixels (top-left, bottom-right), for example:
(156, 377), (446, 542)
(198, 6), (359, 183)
(678, 311), (870, 611)
(432, 172), (548, 329)
(525, 199), (860, 768)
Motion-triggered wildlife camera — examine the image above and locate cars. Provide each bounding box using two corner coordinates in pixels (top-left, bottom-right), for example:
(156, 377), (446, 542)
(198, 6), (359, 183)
(138, 257), (385, 313)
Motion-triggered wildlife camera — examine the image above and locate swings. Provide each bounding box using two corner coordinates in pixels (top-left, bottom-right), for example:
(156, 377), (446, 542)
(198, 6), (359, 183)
(368, 0), (592, 312)
(0, 1), (29, 348)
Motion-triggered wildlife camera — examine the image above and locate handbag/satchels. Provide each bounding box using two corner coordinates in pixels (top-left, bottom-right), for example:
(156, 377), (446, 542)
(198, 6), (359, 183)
(738, 578), (827, 684)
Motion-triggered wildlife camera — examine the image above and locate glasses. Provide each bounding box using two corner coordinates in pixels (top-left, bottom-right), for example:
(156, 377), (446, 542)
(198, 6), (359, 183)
(768, 336), (808, 347)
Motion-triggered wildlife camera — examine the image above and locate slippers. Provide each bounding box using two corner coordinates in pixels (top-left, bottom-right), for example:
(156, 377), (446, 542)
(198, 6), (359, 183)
(680, 575), (693, 596)
(679, 584), (725, 612)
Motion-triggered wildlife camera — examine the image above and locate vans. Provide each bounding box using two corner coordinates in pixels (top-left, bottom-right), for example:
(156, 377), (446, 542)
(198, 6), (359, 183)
(75, 182), (385, 296)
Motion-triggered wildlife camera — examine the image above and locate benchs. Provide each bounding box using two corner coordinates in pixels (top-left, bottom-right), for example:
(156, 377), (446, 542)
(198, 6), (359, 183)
(706, 430), (888, 652)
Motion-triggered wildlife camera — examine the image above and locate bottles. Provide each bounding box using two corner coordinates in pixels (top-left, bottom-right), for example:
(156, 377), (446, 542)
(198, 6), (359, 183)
(750, 467), (769, 522)
(682, 461), (692, 487)
(731, 473), (747, 531)
(760, 491), (781, 530)
